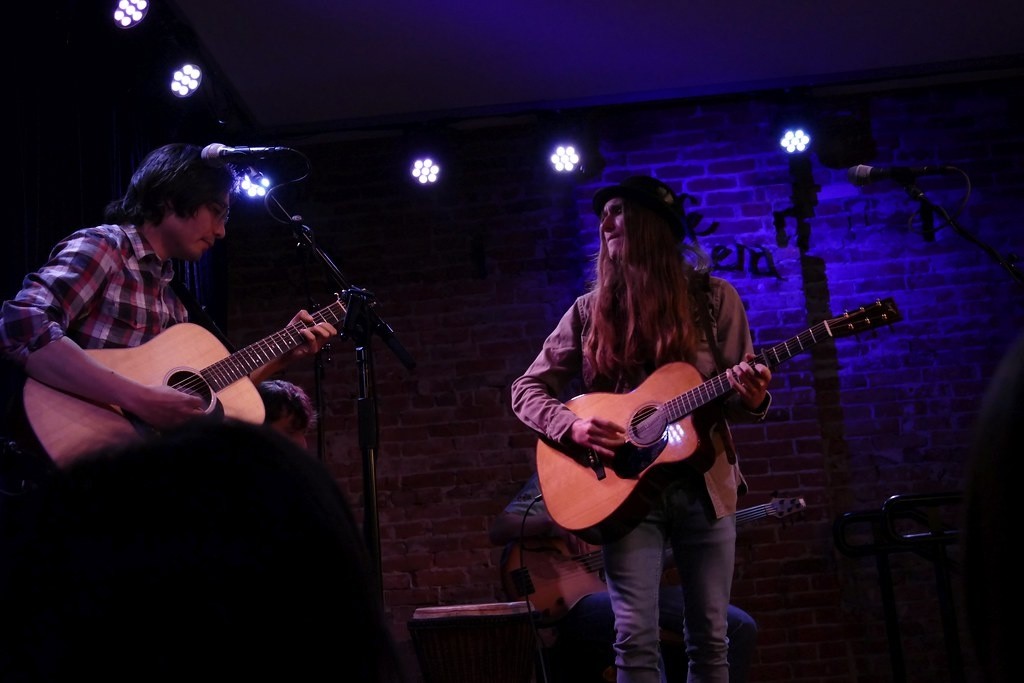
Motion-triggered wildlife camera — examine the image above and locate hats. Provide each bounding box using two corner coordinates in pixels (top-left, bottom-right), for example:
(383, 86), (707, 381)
(591, 176), (685, 244)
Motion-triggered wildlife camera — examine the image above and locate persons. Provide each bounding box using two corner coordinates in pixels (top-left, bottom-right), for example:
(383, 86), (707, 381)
(2, 143), (376, 677)
(501, 175), (772, 683)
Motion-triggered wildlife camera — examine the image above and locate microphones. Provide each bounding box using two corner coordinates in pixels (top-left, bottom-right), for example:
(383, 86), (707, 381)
(201, 143), (287, 168)
(847, 164), (956, 187)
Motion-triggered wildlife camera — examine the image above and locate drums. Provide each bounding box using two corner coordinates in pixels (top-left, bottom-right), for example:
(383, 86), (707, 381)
(405, 599), (542, 683)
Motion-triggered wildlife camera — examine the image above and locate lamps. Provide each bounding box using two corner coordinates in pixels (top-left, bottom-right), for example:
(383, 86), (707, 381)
(406, 132), (445, 186)
(100, 0), (151, 32)
(774, 101), (813, 155)
(547, 139), (609, 183)
(147, 44), (204, 100)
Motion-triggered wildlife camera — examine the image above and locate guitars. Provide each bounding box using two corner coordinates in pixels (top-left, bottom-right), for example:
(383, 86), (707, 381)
(12, 288), (379, 484)
(498, 489), (809, 630)
(534, 296), (906, 547)
